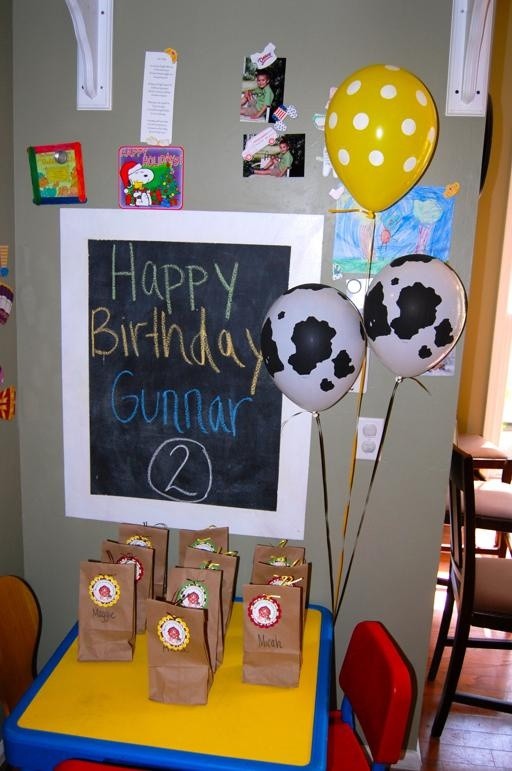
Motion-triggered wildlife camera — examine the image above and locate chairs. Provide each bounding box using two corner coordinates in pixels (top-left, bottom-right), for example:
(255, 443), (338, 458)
(435, 480), (512, 584)
(454, 432), (512, 548)
(0, 574), (43, 720)
(55, 758), (151, 771)
(428, 444), (512, 738)
(327, 621), (418, 771)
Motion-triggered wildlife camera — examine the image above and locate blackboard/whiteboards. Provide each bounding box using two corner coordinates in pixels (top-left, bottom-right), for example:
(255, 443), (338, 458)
(58, 208), (324, 540)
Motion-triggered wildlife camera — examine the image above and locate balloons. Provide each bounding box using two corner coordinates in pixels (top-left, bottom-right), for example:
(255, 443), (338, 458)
(325, 63), (439, 220)
(259, 283), (368, 419)
(363, 254), (468, 383)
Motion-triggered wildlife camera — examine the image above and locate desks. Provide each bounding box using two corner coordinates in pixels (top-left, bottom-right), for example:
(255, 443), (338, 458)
(3, 595), (332, 771)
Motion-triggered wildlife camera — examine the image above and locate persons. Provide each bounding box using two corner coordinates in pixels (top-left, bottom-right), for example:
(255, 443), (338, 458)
(241, 72), (275, 120)
(250, 140), (294, 176)
